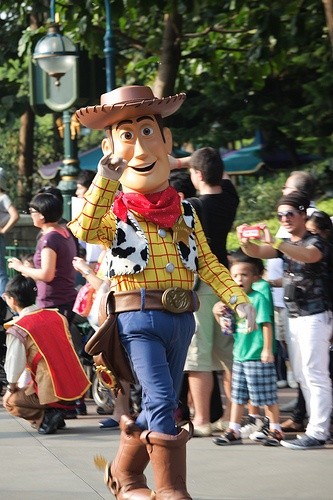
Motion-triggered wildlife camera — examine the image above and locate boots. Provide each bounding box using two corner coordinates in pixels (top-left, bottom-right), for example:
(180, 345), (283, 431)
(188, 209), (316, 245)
(140, 430), (193, 500)
(106, 415), (156, 500)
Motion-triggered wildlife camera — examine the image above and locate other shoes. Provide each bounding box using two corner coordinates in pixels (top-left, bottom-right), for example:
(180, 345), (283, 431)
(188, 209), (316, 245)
(280, 419), (304, 432)
(98, 418), (119, 429)
(97, 402), (135, 414)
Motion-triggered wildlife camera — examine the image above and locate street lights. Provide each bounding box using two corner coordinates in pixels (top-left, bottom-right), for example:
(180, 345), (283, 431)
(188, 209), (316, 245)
(33, 0), (116, 94)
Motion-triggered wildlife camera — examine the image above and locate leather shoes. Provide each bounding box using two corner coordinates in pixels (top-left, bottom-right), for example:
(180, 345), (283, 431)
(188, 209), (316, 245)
(39, 410), (65, 433)
(112, 287), (200, 313)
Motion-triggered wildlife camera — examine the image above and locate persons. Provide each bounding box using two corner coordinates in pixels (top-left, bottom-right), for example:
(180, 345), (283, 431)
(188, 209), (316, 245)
(70, 170), (133, 428)
(166, 147), (240, 436)
(235, 191), (332, 449)
(169, 172), (225, 426)
(3, 275), (93, 434)
(212, 261), (286, 447)
(0, 166), (20, 295)
(265, 171), (318, 432)
(232, 246), (274, 440)
(8, 193), (79, 419)
(305, 211), (332, 378)
(66, 85), (258, 500)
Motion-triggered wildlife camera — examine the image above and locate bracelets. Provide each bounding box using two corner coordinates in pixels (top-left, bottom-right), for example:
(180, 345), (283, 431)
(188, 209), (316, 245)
(272, 239), (283, 250)
(240, 242), (251, 249)
(6, 387), (15, 393)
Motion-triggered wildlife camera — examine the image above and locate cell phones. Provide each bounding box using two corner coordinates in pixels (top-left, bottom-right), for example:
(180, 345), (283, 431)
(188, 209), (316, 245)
(240, 226), (260, 240)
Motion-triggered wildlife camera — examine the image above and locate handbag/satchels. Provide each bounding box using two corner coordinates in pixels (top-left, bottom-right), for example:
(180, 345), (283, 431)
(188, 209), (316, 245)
(84, 291), (137, 399)
(72, 283), (95, 317)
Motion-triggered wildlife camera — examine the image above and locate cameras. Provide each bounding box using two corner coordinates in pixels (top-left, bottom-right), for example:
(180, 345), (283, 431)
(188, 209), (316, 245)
(283, 284), (304, 318)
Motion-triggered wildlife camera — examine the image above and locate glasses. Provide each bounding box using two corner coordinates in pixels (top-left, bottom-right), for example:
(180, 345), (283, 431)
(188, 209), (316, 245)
(282, 186), (292, 190)
(29, 208), (38, 213)
(2, 293), (9, 301)
(277, 212), (301, 218)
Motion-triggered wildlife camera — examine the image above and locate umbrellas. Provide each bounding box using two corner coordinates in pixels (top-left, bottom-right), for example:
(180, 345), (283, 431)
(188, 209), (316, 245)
(39, 139), (273, 174)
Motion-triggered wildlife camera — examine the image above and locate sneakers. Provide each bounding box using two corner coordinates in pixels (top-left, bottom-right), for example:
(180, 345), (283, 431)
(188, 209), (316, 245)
(263, 429), (284, 446)
(239, 424), (259, 438)
(249, 431), (267, 441)
(212, 419), (228, 430)
(212, 431), (242, 446)
(181, 423), (211, 436)
(280, 434), (325, 449)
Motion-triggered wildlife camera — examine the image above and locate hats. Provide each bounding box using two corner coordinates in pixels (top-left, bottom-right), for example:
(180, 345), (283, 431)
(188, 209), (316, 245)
(276, 191), (310, 210)
(0, 167), (10, 191)
(75, 86), (185, 129)
